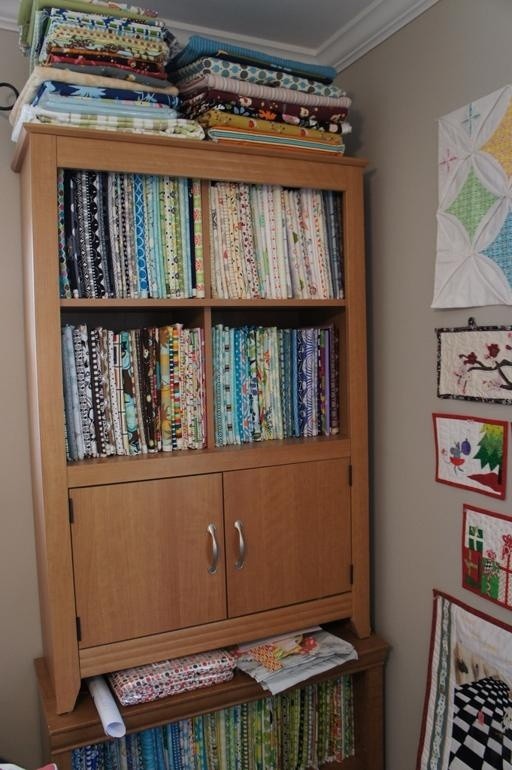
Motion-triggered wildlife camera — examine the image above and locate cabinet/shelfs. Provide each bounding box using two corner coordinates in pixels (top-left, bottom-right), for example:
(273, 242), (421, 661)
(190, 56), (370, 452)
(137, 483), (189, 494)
(32, 635), (394, 770)
(11, 124), (376, 716)
